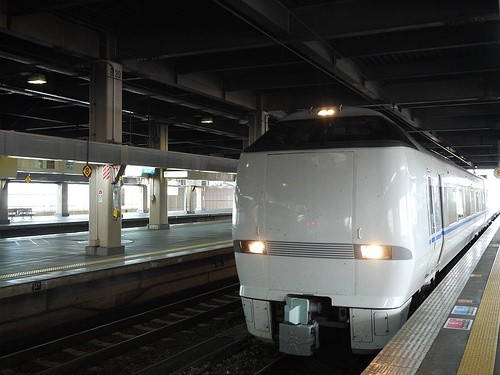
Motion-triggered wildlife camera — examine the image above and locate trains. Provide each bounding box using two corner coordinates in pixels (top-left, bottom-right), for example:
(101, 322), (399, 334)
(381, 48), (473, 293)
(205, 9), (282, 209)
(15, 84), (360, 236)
(231, 100), (500, 356)
